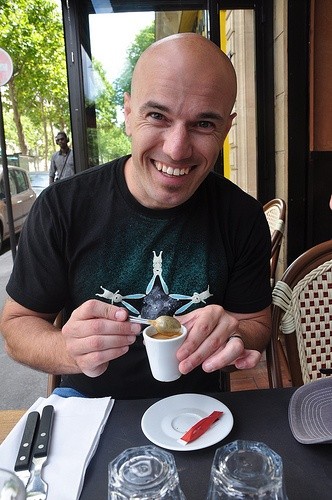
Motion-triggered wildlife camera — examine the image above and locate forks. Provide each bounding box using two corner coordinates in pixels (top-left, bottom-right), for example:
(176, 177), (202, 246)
(23, 405), (54, 500)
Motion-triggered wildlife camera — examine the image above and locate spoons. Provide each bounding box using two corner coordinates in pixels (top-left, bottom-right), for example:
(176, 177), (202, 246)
(126, 315), (181, 336)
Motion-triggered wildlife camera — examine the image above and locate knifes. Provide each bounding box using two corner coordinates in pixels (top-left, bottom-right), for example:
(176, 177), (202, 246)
(12, 411), (40, 500)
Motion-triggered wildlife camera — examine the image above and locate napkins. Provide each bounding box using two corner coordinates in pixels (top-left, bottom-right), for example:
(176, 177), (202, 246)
(0, 393), (115, 500)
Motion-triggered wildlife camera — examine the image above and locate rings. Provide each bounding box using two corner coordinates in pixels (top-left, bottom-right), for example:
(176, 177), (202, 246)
(227, 336), (244, 345)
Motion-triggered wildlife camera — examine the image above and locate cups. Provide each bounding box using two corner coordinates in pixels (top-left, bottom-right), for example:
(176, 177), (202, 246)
(142, 324), (188, 383)
(107, 444), (187, 500)
(206, 439), (288, 500)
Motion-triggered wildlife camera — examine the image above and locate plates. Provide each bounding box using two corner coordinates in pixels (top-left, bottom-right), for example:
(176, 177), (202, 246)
(139, 393), (234, 452)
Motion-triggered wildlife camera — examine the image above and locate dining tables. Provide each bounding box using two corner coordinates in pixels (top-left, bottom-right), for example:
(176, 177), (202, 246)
(79, 389), (332, 500)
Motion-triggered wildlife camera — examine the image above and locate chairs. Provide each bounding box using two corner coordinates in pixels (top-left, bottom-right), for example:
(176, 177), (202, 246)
(269, 241), (332, 390)
(263, 198), (287, 289)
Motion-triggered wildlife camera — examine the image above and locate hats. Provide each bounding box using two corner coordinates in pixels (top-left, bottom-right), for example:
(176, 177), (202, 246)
(287, 375), (332, 445)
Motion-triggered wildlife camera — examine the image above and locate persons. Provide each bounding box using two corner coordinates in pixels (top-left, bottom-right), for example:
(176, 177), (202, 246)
(49, 132), (75, 184)
(0, 32), (272, 397)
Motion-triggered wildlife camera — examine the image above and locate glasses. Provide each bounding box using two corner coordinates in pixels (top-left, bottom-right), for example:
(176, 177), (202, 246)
(56, 137), (65, 142)
